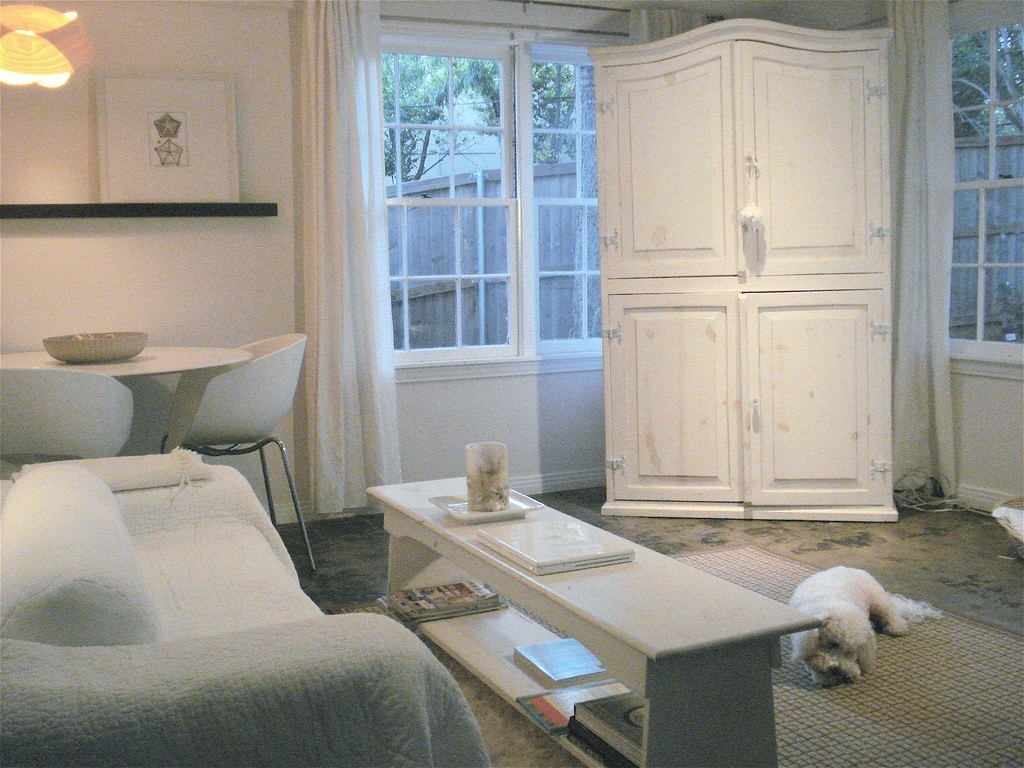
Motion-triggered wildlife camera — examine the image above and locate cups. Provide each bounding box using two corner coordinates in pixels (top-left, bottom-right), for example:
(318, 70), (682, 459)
(466, 442), (509, 511)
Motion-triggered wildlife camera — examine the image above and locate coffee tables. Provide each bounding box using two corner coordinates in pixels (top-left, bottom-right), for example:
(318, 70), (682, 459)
(366, 475), (822, 768)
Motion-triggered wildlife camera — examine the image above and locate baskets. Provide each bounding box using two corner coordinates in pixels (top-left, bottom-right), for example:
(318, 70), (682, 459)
(42, 331), (149, 365)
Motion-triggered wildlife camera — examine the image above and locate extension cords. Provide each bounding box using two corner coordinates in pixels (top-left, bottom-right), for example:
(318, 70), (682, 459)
(893, 490), (961, 507)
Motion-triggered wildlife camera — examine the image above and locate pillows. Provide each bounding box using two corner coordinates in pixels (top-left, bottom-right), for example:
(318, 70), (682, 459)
(0, 465), (166, 645)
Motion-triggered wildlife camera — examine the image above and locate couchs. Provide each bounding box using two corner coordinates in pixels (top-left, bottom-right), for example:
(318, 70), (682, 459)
(0, 447), (492, 768)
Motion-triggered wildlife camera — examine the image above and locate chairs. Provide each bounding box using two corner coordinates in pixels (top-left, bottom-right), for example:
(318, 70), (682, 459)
(184, 333), (316, 570)
(0, 367), (135, 460)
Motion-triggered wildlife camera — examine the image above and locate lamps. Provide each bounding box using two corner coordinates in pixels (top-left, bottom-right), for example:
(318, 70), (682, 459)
(0, 5), (97, 87)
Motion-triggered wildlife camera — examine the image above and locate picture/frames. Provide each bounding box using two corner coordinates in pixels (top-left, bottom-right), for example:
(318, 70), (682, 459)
(93, 64), (241, 204)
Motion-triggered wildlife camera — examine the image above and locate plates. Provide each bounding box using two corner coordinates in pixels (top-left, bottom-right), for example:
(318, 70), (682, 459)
(43, 332), (148, 362)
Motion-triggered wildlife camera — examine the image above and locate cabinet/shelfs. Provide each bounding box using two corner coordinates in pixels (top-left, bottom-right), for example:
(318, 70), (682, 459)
(587, 17), (900, 522)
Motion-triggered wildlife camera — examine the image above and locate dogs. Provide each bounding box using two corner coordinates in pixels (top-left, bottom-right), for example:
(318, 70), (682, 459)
(787, 565), (945, 686)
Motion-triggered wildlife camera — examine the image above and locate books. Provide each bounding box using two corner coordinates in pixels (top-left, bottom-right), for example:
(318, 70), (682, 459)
(558, 690), (646, 768)
(516, 678), (633, 734)
(513, 637), (614, 687)
(377, 581), (508, 626)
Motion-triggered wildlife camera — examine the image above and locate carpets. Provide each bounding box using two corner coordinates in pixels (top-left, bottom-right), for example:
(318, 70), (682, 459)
(328, 543), (1024, 768)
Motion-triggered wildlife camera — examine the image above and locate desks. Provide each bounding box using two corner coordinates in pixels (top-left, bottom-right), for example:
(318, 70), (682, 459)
(0, 347), (253, 457)
